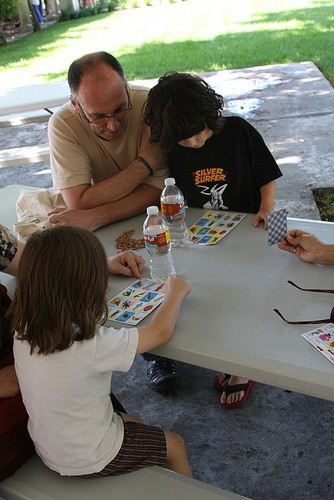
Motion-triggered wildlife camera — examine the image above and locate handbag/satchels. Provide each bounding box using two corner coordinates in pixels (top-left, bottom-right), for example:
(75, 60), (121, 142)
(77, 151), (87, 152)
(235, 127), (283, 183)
(13, 186), (67, 245)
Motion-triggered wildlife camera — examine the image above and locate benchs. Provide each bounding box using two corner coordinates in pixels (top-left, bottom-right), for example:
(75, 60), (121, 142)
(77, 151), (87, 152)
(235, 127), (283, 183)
(0, 449), (249, 500)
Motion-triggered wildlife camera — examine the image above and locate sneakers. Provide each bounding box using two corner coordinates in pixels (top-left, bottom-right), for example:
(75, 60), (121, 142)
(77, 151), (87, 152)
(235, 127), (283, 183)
(143, 358), (181, 394)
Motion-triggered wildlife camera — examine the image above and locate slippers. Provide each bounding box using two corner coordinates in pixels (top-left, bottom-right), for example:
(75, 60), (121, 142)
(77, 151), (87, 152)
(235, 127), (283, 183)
(214, 372), (255, 409)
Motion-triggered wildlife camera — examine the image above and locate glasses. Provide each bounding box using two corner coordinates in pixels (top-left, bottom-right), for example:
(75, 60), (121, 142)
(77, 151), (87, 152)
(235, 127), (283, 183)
(76, 85), (133, 125)
(273, 280), (334, 324)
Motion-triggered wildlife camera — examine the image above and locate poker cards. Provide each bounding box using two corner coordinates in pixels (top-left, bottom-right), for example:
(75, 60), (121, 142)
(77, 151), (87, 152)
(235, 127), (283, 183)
(266, 208), (289, 247)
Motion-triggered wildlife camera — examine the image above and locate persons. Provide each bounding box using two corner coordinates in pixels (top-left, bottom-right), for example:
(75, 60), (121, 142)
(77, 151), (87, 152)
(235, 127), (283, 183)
(49, 52), (182, 388)
(32, 0), (44, 24)
(278, 229), (334, 264)
(144, 72), (283, 407)
(13, 225), (193, 478)
(0, 225), (146, 477)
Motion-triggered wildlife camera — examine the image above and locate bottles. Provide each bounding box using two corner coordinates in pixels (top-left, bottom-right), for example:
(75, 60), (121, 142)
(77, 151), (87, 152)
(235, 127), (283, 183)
(160, 177), (189, 248)
(142, 206), (176, 282)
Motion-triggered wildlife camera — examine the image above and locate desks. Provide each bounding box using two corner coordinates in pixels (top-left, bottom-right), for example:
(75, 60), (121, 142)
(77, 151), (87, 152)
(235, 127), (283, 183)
(0, 185), (334, 401)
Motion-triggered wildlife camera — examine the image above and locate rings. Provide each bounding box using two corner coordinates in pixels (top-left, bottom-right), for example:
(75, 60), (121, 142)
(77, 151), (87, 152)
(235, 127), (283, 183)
(294, 248), (296, 253)
